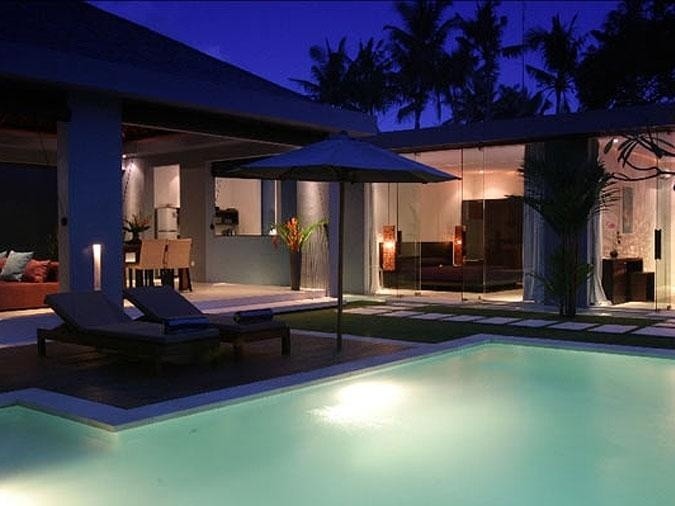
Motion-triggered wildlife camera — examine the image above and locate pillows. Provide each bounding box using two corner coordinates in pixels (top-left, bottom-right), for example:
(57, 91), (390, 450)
(0, 250), (51, 283)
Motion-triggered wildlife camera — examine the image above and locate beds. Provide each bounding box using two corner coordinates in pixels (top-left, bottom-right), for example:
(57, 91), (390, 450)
(36, 284), (290, 384)
(380, 241), (523, 292)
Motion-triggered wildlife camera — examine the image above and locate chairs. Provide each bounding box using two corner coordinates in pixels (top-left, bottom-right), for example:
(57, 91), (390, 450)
(124, 238), (192, 291)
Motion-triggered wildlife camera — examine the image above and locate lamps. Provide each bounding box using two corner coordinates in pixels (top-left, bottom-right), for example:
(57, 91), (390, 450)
(383, 226), (396, 271)
(454, 226), (463, 266)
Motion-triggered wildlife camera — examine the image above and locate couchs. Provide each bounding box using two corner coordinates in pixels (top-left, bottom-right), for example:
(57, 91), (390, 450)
(0, 261), (59, 311)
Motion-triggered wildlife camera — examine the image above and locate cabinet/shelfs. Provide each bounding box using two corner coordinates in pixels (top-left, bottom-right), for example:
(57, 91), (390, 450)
(603, 258), (642, 304)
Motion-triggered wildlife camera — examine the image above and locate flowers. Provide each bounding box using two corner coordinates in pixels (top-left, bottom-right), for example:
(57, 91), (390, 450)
(268, 216), (330, 252)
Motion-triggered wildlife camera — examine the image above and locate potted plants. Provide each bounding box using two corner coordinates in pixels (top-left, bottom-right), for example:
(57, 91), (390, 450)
(604, 230), (622, 258)
(121, 211), (152, 245)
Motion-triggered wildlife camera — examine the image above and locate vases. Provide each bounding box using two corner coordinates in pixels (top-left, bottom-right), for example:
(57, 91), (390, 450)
(290, 252), (302, 290)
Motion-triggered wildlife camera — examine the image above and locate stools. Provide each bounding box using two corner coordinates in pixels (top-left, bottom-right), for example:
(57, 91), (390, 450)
(630, 272), (654, 302)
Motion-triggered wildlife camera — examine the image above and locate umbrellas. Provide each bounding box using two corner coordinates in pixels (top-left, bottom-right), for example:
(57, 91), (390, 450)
(240, 129), (464, 347)
